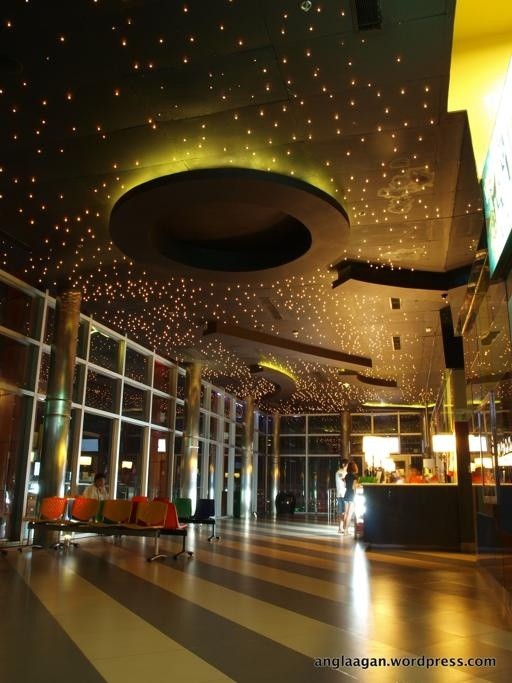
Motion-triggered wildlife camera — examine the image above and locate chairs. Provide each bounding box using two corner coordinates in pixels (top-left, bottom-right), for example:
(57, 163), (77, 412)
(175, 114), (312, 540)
(17, 495), (221, 563)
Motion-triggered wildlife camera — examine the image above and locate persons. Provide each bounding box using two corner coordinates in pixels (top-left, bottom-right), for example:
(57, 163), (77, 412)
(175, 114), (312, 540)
(389, 466), (441, 483)
(335, 459), (349, 533)
(84, 474), (126, 544)
(343, 461), (362, 535)
